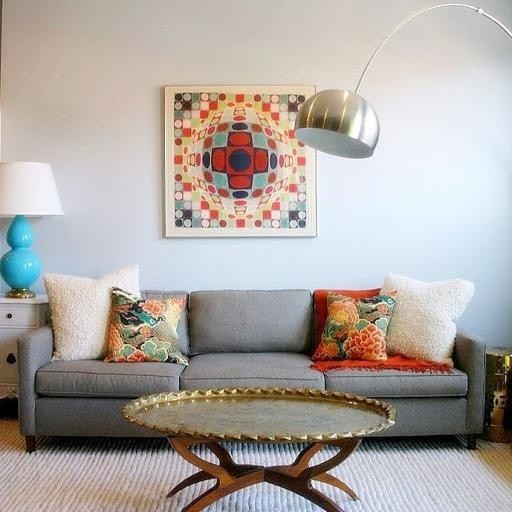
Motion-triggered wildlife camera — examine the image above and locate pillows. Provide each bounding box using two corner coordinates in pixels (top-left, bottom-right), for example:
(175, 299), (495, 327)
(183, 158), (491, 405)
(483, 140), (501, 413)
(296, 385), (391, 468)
(43, 264), (142, 363)
(103, 286), (190, 367)
(312, 290), (397, 361)
(380, 274), (473, 368)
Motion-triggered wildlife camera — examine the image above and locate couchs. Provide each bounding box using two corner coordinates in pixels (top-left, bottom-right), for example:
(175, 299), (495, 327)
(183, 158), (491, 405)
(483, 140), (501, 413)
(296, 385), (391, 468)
(15, 289), (486, 452)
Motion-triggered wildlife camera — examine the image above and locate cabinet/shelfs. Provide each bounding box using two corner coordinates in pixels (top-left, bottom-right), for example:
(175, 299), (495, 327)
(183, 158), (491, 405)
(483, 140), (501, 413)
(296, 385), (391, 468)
(0, 296), (49, 399)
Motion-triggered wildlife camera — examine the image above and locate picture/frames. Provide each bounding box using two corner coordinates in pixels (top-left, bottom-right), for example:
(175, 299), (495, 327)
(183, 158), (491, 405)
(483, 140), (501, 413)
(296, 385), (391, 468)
(164, 85), (316, 239)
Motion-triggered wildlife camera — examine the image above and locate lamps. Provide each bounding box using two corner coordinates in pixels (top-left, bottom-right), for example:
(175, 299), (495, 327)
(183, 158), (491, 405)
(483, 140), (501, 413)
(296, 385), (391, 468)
(295, 1), (512, 158)
(0, 161), (64, 298)
